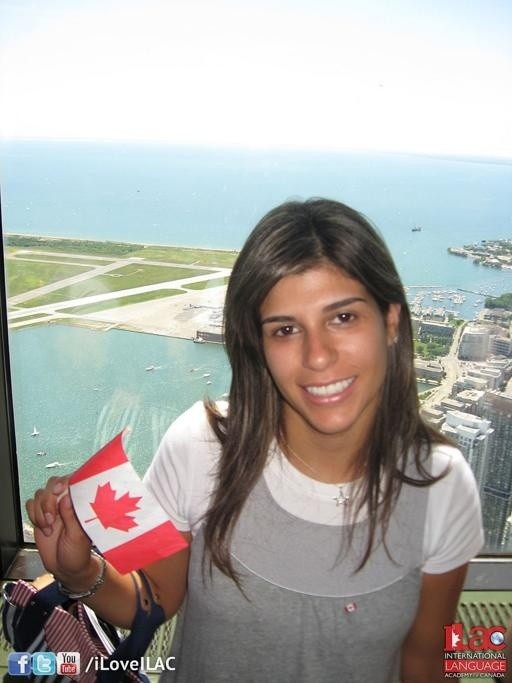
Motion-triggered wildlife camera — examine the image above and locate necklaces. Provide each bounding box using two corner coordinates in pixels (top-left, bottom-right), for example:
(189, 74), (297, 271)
(278, 427), (352, 506)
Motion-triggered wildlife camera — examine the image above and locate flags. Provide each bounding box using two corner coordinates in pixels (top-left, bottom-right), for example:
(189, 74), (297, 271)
(69, 425), (189, 576)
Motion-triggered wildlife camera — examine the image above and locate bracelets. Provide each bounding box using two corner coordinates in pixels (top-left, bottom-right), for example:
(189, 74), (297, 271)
(58, 551), (107, 600)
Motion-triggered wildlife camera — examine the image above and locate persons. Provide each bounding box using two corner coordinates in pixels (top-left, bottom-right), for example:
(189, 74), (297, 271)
(20, 196), (488, 682)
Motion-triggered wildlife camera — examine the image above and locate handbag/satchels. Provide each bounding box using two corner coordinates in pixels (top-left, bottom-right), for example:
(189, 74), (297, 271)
(1, 537), (175, 683)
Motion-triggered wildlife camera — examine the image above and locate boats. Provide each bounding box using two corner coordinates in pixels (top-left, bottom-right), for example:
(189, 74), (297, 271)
(146, 361), (216, 388)
(410, 225), (422, 232)
(27, 423), (62, 468)
